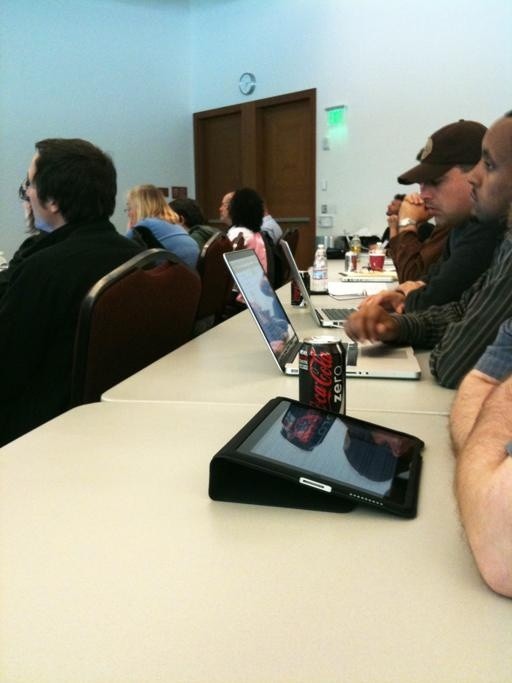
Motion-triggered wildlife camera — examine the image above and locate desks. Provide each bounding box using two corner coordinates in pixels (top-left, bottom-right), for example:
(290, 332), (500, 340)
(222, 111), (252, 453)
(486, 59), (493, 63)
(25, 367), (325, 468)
(0, 256), (512, 683)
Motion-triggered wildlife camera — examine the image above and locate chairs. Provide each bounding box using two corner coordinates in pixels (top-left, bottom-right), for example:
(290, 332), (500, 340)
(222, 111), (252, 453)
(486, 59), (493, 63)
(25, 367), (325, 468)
(72, 248), (201, 405)
(191, 227), (299, 334)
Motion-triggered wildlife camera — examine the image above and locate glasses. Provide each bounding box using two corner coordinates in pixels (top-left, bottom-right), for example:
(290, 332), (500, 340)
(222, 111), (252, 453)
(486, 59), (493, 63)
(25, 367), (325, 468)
(123, 209), (129, 213)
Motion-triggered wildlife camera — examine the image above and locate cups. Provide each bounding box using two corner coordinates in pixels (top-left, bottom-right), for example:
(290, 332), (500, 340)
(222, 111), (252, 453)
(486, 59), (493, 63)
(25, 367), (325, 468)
(369, 253), (385, 272)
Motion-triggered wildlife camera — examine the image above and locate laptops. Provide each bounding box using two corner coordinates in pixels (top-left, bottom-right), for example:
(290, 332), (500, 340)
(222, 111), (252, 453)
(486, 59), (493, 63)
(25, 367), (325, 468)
(223, 248), (422, 380)
(279, 239), (360, 328)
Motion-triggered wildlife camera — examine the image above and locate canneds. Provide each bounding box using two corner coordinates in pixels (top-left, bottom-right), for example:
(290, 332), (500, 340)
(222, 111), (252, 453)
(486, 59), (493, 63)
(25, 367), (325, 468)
(323, 234), (335, 257)
(289, 269), (311, 307)
(344, 251), (358, 273)
(297, 334), (348, 418)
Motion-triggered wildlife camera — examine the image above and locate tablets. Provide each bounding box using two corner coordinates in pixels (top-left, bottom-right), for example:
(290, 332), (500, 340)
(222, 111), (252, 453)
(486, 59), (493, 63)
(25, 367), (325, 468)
(209, 396), (425, 519)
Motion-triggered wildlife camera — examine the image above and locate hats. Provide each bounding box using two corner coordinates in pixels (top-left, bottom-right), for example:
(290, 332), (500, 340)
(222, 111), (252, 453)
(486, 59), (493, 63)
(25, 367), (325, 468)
(397, 120), (488, 185)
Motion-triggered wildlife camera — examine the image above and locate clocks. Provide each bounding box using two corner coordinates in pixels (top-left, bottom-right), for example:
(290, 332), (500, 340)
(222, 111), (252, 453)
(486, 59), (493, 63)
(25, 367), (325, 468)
(238, 70), (256, 96)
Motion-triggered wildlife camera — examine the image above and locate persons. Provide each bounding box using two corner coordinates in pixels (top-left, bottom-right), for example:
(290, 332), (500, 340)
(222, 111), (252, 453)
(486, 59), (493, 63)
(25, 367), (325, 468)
(335, 416), (418, 482)
(260, 203), (284, 247)
(168, 198), (225, 255)
(342, 111), (511, 390)
(358, 119), (506, 314)
(219, 191), (236, 234)
(447, 317), (511, 601)
(368, 192), (450, 284)
(125, 184), (200, 272)
(225, 188), (267, 304)
(2, 138), (147, 446)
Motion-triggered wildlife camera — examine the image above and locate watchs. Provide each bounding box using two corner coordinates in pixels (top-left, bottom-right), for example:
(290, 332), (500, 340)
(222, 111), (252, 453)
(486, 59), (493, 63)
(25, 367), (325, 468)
(398, 218), (416, 226)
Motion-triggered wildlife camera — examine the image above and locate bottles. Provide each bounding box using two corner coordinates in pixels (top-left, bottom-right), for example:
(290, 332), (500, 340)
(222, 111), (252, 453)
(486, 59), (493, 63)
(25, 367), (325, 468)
(313, 252), (328, 290)
(315, 242), (326, 260)
(349, 234), (361, 258)
